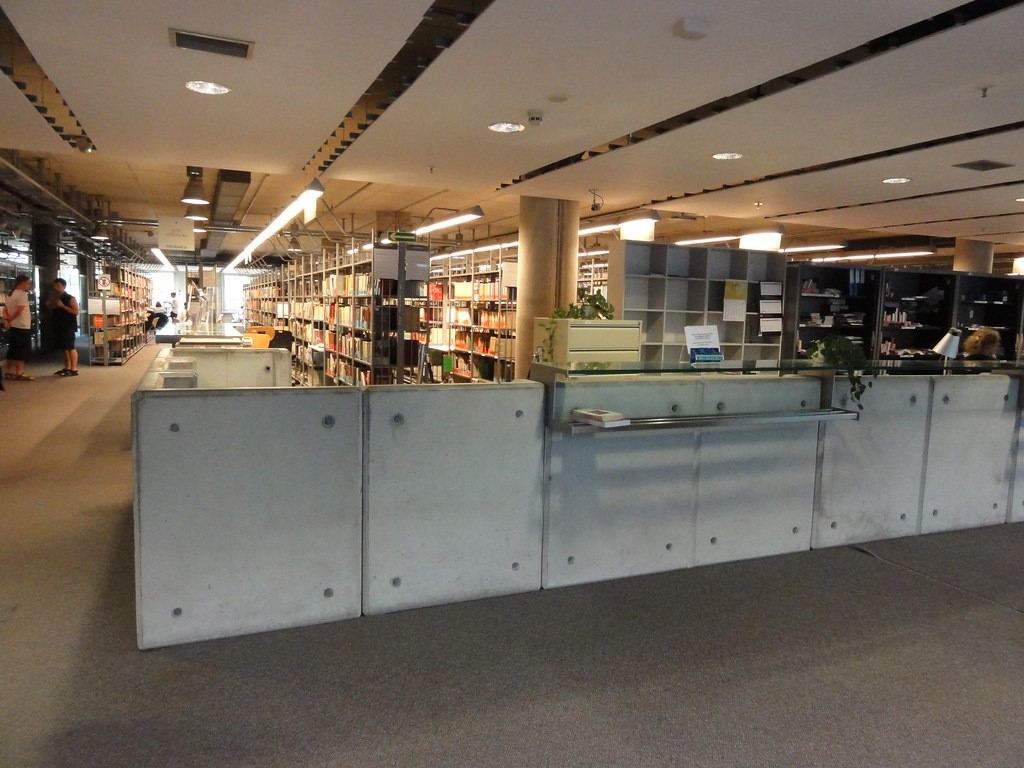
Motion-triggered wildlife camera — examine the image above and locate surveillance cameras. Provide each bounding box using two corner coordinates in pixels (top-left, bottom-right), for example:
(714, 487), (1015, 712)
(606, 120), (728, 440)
(591, 204), (600, 211)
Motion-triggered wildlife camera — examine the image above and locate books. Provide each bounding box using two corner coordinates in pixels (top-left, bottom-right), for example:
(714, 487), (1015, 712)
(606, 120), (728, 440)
(354, 337), (372, 363)
(354, 272), (372, 296)
(478, 283), (510, 301)
(797, 268), (869, 355)
(353, 306), (370, 329)
(880, 282), (940, 358)
(241, 286), (276, 326)
(93, 270), (152, 311)
(289, 274), (352, 385)
(571, 407), (630, 428)
(93, 312), (151, 358)
(479, 308), (512, 328)
(418, 281), (496, 381)
(354, 367), (370, 386)
(592, 272), (608, 303)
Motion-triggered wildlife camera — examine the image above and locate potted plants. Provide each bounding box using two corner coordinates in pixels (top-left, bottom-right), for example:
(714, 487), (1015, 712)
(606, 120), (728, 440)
(537, 285), (616, 361)
(807, 335), (880, 409)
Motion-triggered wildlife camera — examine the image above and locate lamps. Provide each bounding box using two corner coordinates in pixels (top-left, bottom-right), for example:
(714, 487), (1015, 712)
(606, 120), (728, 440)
(932, 327), (962, 375)
(90, 215), (109, 240)
(151, 180), (938, 272)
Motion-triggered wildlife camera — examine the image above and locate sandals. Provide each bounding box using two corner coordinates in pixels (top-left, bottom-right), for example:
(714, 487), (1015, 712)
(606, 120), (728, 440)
(15, 372), (34, 380)
(4, 372), (16, 380)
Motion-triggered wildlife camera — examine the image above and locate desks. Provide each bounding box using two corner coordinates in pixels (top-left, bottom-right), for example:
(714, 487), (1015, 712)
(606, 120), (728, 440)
(156, 322), (243, 347)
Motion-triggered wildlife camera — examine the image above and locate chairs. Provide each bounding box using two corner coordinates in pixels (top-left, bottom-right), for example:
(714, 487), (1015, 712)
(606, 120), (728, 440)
(242, 334), (270, 348)
(248, 325), (276, 342)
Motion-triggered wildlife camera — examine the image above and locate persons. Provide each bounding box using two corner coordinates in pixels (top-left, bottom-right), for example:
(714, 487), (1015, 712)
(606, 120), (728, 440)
(1, 275), (36, 380)
(169, 293), (178, 323)
(155, 301), (167, 315)
(47, 278), (79, 376)
(952, 327), (1000, 375)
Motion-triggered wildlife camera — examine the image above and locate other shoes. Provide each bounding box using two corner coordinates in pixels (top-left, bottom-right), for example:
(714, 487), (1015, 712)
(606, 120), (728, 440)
(54, 369), (79, 376)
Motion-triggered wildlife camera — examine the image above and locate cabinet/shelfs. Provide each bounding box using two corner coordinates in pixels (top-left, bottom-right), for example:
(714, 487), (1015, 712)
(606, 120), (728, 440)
(244, 229), (1024, 388)
(87, 261), (154, 366)
